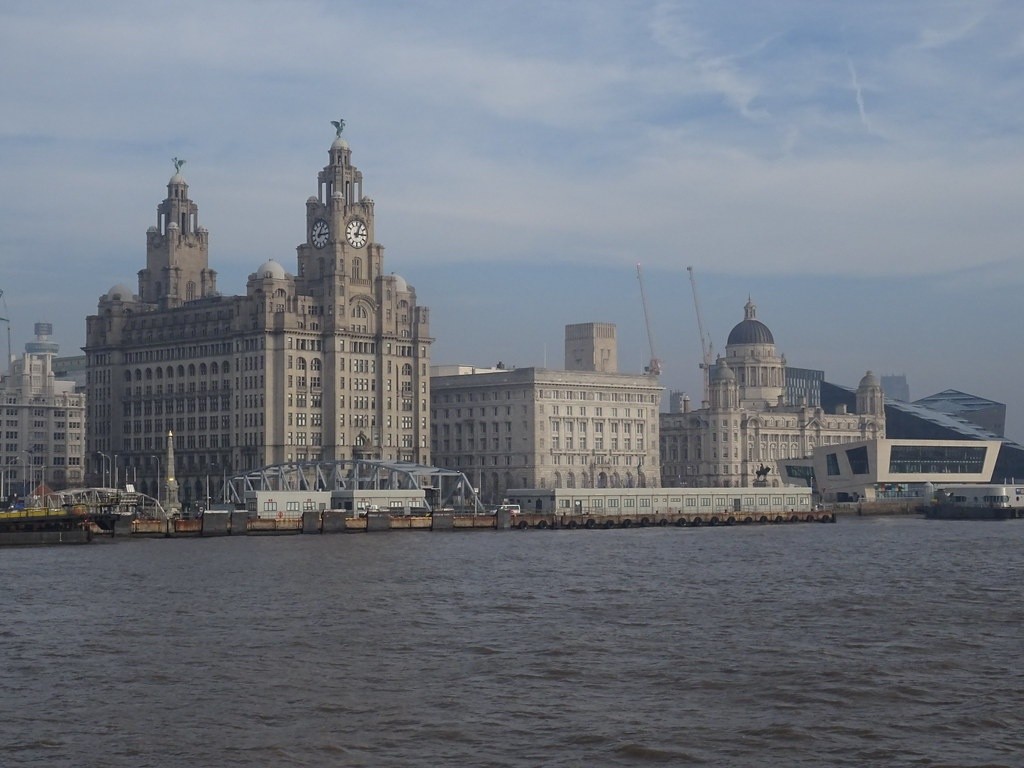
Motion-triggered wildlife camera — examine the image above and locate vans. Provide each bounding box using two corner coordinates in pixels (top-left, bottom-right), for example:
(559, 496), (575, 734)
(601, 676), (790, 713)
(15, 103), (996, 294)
(489, 505), (521, 517)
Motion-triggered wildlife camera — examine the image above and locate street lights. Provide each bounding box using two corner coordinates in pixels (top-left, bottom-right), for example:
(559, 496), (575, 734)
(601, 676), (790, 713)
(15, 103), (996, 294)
(7, 450), (48, 507)
(152, 455), (160, 503)
(102, 454), (111, 489)
(206, 462), (215, 510)
(97, 450), (105, 488)
(114, 454), (118, 487)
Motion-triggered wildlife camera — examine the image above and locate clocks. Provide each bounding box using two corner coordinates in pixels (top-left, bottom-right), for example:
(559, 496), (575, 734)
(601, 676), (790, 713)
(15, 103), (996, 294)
(311, 220), (329, 249)
(345, 220), (368, 249)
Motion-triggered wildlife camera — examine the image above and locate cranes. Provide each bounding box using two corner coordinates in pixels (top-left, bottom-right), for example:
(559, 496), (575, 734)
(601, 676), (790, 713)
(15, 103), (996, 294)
(635, 260), (662, 376)
(687, 265), (715, 369)
